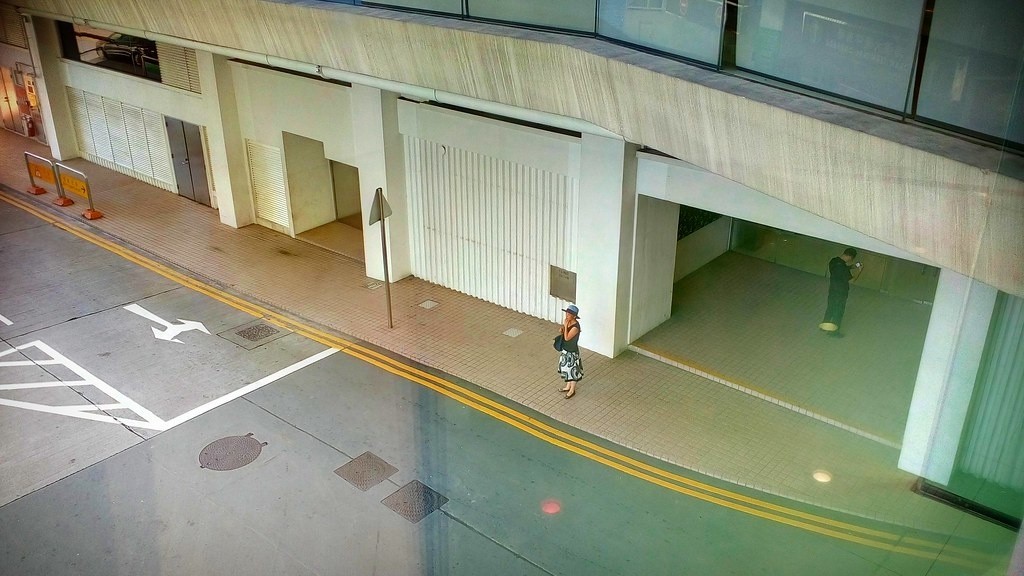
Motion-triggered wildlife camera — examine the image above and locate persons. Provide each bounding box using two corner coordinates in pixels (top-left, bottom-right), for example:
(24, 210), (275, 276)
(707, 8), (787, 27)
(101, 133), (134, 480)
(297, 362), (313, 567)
(820, 247), (863, 338)
(552, 305), (585, 400)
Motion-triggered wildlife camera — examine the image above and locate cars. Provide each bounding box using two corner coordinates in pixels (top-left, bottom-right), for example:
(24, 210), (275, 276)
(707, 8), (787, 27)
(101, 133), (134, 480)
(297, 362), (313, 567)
(95, 32), (157, 67)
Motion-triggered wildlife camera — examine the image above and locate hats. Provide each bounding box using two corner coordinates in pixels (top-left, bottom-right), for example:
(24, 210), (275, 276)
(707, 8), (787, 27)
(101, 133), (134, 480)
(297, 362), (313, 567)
(561, 305), (581, 319)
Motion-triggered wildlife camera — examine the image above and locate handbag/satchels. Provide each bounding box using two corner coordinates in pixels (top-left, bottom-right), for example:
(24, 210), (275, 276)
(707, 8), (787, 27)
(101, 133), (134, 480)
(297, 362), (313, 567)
(553, 333), (564, 352)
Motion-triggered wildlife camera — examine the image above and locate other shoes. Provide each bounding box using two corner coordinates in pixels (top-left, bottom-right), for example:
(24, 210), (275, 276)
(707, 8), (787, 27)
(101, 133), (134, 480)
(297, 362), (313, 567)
(558, 387), (570, 392)
(564, 390), (575, 399)
(827, 333), (844, 338)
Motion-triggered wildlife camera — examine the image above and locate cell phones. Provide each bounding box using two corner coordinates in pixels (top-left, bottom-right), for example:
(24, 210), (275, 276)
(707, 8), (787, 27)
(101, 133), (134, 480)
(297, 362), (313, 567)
(855, 262), (862, 268)
